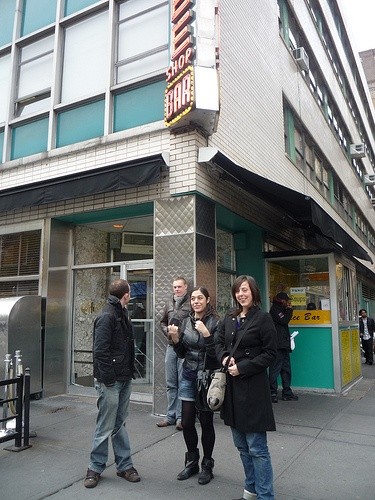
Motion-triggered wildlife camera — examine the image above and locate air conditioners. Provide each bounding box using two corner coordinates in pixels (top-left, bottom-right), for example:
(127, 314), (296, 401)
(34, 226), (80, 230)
(293, 47), (309, 70)
(351, 143), (367, 158)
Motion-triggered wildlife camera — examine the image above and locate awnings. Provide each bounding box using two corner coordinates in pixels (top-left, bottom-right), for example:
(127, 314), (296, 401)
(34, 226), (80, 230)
(0, 149), (170, 211)
(198, 146), (374, 265)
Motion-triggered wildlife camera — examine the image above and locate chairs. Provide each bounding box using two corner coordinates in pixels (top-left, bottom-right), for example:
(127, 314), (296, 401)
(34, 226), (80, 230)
(134, 339), (151, 382)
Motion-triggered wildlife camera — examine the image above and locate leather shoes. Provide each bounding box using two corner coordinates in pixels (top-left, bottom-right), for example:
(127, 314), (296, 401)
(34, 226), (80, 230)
(157, 414), (185, 433)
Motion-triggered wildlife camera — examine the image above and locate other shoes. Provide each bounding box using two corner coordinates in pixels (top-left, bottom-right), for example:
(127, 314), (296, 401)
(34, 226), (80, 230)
(84, 462), (143, 488)
(270, 390), (297, 404)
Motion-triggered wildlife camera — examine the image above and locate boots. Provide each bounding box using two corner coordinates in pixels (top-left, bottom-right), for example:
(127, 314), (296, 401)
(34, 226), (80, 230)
(241, 487), (273, 499)
(176, 448), (218, 487)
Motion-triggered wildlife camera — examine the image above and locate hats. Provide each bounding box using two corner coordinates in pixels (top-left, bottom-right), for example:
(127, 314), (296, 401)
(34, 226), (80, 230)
(275, 291), (292, 300)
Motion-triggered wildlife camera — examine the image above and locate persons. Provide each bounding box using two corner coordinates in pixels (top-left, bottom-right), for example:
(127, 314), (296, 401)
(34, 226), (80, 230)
(168, 287), (221, 485)
(156, 277), (194, 430)
(85, 279), (141, 488)
(269, 292), (299, 403)
(214, 275), (278, 500)
(359, 309), (375, 365)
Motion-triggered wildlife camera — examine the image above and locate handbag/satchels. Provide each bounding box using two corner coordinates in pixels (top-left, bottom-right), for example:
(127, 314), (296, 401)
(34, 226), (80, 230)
(197, 367), (226, 411)
